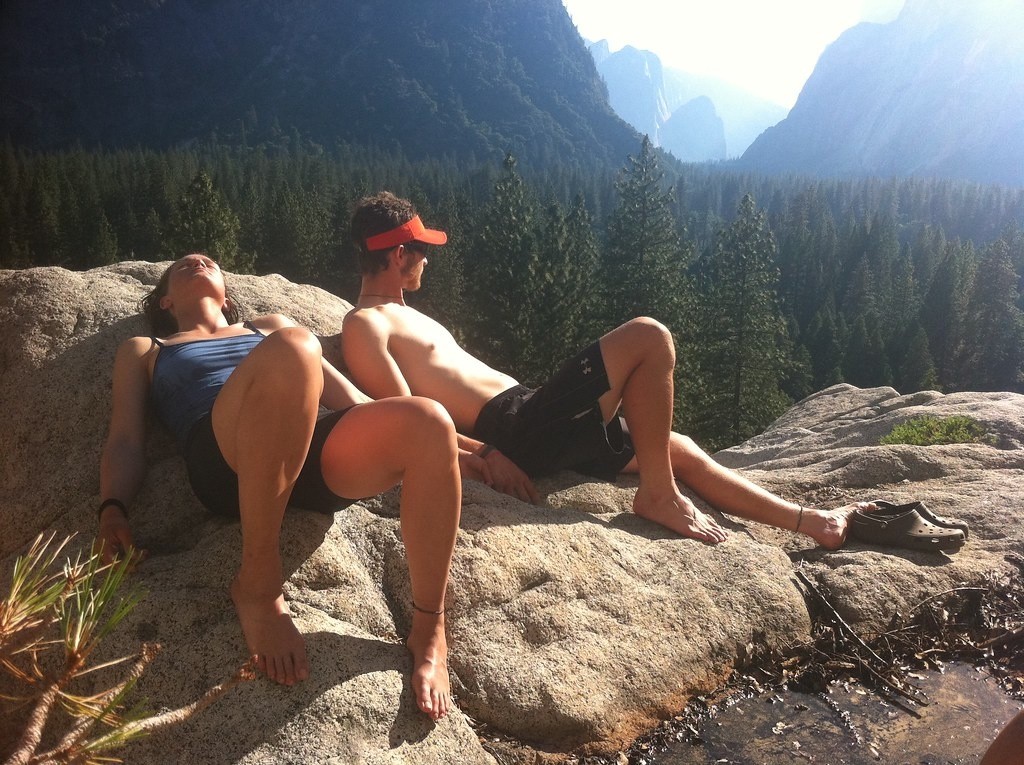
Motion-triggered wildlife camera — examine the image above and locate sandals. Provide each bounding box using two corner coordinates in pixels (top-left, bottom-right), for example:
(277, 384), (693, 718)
(870, 499), (969, 538)
(850, 509), (966, 551)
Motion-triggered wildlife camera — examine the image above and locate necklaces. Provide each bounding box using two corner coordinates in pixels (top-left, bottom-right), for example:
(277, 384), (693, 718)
(359, 295), (402, 298)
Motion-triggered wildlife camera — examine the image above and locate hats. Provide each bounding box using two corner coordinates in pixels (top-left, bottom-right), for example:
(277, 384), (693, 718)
(365, 215), (447, 251)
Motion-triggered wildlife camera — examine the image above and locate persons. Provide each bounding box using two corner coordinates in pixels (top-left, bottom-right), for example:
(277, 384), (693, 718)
(341, 191), (877, 549)
(97, 254), (494, 719)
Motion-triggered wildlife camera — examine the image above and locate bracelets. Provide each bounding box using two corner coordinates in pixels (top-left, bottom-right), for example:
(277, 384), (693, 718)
(98, 499), (129, 522)
(479, 444), (496, 458)
